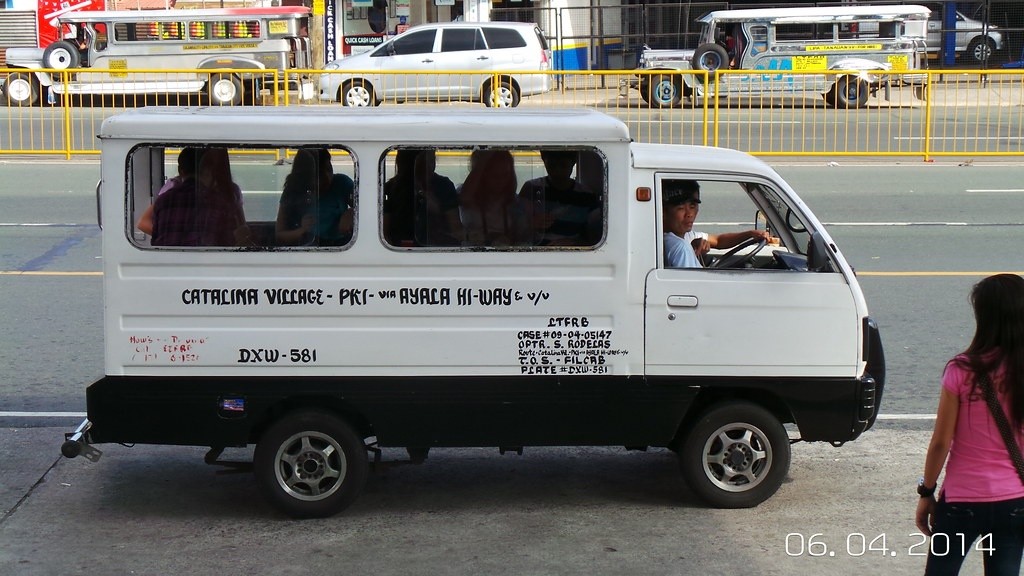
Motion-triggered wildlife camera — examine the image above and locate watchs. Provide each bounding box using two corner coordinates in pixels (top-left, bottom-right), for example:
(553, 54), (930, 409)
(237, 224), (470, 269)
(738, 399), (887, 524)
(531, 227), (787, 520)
(917, 476), (937, 497)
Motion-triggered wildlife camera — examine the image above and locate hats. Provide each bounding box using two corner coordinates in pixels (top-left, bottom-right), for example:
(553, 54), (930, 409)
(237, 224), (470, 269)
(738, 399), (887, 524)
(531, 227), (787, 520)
(663, 180), (701, 207)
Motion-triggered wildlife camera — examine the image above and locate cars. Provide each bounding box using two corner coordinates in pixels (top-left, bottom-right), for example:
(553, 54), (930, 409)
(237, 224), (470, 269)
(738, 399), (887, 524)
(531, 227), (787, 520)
(314, 20), (557, 108)
(926, 2), (1004, 62)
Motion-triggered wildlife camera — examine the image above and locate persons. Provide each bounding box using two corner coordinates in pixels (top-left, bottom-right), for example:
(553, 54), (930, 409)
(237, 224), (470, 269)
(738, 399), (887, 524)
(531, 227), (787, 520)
(917, 273), (1024, 576)
(138, 146), (256, 246)
(275, 148), (353, 247)
(394, 15), (407, 34)
(79, 37), (89, 50)
(723, 32), (736, 62)
(661, 180), (773, 269)
(383, 148), (603, 247)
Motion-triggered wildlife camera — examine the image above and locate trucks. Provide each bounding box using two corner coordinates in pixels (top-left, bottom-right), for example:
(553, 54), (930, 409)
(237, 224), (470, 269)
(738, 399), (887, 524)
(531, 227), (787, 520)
(624, 4), (932, 109)
(1, 3), (312, 106)
(59, 102), (886, 511)
